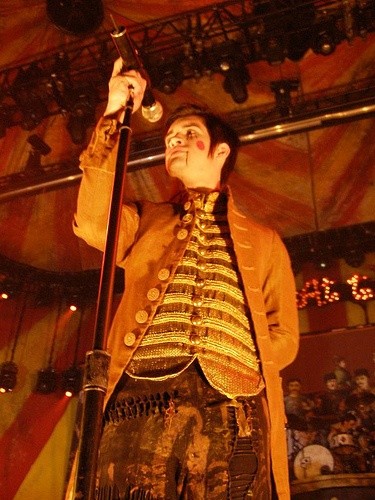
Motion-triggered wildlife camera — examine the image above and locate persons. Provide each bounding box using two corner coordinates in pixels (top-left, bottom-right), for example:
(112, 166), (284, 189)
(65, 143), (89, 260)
(71, 55), (299, 500)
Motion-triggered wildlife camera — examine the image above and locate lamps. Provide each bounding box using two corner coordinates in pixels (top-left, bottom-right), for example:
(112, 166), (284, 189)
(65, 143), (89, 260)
(0, 303), (32, 395)
(35, 303), (63, 394)
(2, 1), (372, 155)
(64, 310), (85, 398)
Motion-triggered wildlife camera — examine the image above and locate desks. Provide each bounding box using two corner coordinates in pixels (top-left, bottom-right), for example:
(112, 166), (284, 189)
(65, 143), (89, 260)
(289, 472), (375, 500)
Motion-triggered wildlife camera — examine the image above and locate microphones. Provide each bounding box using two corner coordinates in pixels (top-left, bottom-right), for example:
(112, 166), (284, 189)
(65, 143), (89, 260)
(110, 26), (163, 122)
